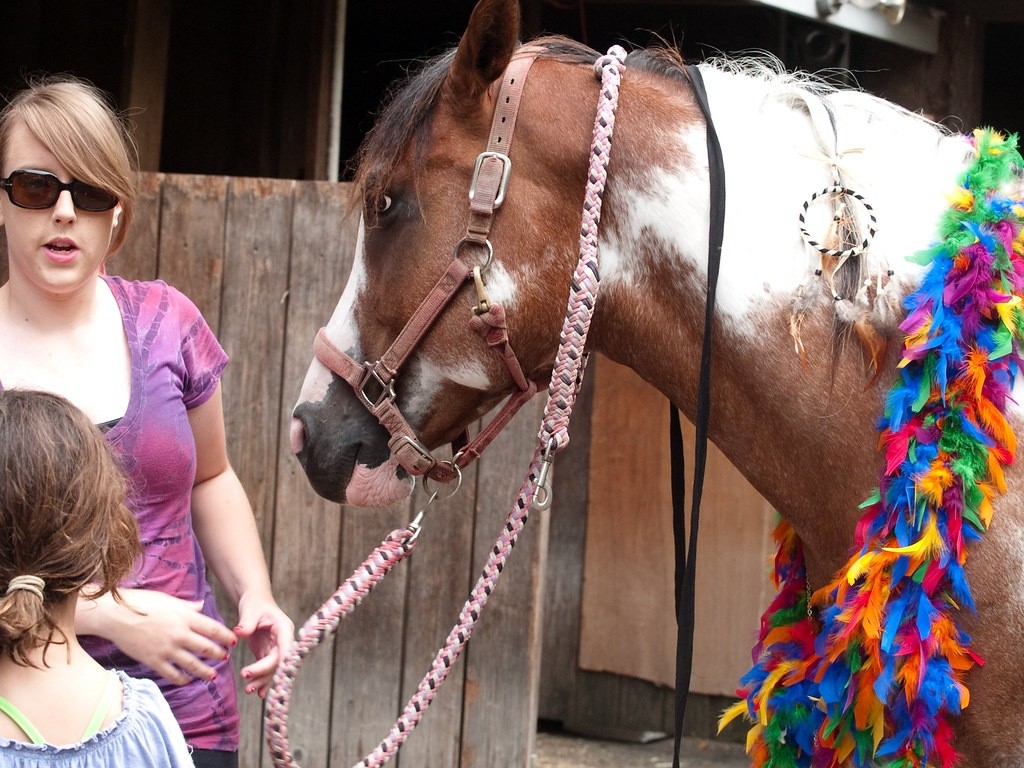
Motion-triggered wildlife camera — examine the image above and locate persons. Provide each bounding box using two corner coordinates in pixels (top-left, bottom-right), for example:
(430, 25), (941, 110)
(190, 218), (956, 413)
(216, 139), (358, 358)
(0, 83), (298, 768)
(0, 389), (195, 768)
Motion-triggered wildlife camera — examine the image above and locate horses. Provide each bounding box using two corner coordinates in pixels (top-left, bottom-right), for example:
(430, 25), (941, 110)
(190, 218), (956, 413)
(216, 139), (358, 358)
(289, 0), (1024, 768)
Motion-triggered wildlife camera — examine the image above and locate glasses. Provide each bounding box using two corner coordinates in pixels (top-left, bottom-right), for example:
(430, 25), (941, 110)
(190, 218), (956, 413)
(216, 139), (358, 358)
(0, 170), (120, 212)
